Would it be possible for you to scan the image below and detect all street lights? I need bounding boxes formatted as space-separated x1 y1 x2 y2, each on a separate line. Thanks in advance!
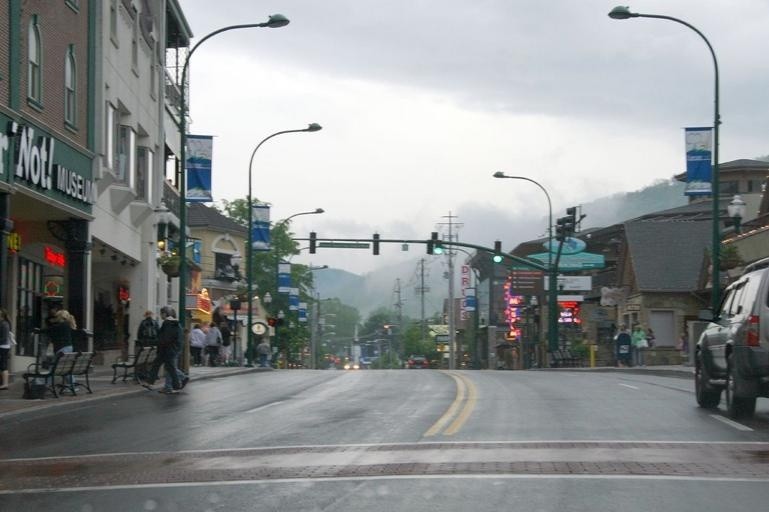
605 4 723 318
728 194 746 234
245 122 323 366
180 12 292 375
302 264 327 275
384 323 401 329
277 208 326 360
492 171 556 360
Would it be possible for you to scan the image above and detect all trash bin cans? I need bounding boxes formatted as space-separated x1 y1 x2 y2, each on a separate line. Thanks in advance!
259 354 267 367
614 332 631 362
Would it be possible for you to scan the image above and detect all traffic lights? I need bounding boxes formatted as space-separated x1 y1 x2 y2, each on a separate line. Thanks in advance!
555 217 566 241
493 242 504 264
565 207 574 232
435 240 442 255
268 317 275 325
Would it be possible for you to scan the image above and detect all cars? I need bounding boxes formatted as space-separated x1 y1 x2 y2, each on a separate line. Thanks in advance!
345 361 359 370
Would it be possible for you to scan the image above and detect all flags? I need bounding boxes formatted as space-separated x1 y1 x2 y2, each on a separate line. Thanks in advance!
466 287 476 313
278 263 292 292
683 126 713 197
185 135 212 202
288 288 300 311
251 206 271 251
298 304 307 322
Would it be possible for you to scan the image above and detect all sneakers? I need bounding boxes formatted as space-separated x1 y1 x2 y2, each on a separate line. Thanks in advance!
141 383 153 390
158 377 189 393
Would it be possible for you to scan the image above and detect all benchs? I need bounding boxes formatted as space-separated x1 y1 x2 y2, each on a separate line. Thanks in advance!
22 351 95 399
111 345 158 385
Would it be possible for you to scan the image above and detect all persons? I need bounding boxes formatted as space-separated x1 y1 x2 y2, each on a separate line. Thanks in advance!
610 322 688 369
43 300 233 392
257 338 270 367
1 308 17 389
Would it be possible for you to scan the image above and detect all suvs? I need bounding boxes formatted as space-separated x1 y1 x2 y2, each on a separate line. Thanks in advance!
407 353 429 370
692 256 768 415
